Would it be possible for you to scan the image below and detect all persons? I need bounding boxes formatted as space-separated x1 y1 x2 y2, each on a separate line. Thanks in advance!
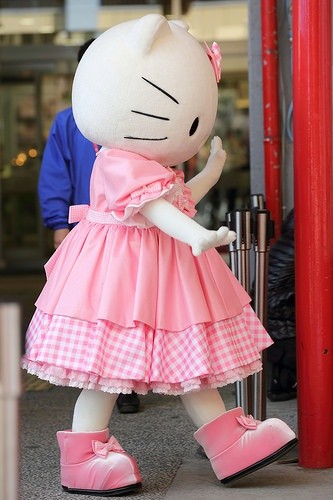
38 38 142 410
249 209 297 401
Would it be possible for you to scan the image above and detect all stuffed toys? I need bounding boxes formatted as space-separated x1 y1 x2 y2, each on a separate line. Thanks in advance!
19 13 299 496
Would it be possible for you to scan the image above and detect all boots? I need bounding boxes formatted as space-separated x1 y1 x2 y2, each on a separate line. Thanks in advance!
194 406 296 484
56 429 140 492
